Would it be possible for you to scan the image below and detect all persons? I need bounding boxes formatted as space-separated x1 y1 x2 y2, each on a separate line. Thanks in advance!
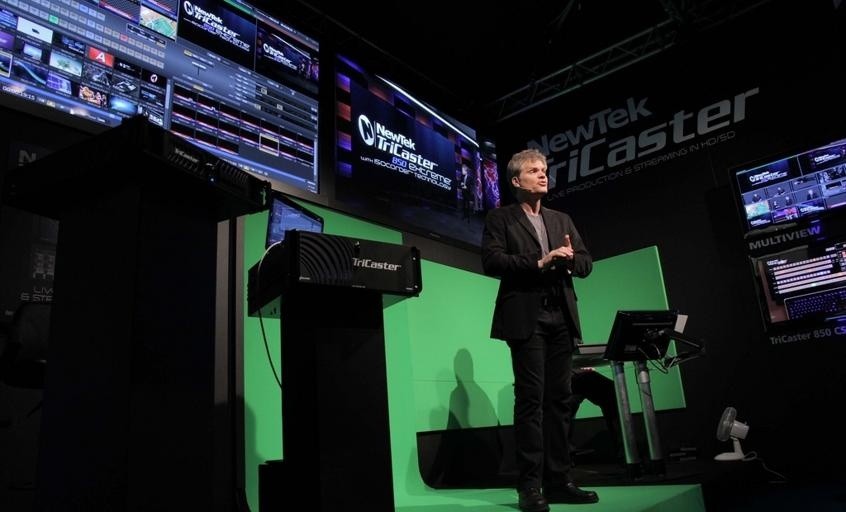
772 200 780 209
785 197 791 206
751 193 765 203
480 147 600 511
457 163 476 226
807 188 819 200
774 186 787 195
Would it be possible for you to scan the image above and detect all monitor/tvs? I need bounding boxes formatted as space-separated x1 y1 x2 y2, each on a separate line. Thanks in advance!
1 1 505 256
727 136 846 232
744 219 845 359
604 308 678 360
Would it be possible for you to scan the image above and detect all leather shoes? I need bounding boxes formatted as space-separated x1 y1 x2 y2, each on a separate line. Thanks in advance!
518 489 550 512
550 482 599 504
